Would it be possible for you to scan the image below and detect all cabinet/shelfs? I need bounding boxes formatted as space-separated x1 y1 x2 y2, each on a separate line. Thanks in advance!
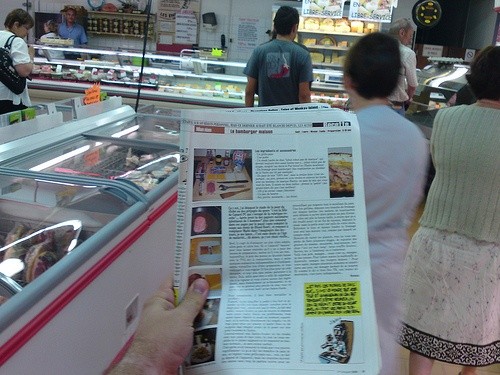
85 10 155 41
283 8 383 75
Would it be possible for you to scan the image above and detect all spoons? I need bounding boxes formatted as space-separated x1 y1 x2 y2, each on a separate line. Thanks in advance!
220 185 244 190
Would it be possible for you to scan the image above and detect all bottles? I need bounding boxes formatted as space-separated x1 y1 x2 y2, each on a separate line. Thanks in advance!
223 151 230 166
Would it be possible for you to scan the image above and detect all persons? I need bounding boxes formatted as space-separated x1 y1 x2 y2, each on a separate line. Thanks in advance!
389 18 419 118
242 6 314 106
106 278 209 375
58 8 88 60
0 8 38 115
258 30 310 52
343 32 429 375
393 46 500 375
36 20 65 61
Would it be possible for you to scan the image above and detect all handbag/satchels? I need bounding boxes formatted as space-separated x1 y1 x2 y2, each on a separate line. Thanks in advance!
0 34 27 95
